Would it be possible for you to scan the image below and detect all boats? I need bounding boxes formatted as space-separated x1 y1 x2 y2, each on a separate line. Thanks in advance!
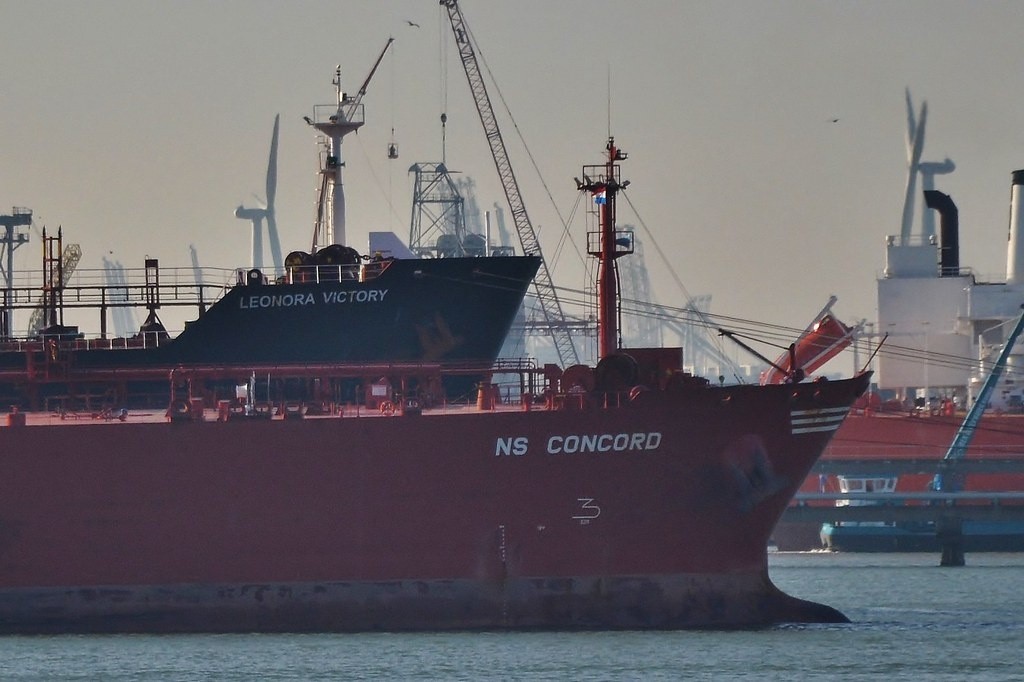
761 86 1024 554
0 35 876 636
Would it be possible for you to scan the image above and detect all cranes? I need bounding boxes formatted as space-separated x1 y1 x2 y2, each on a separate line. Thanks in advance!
444 1 581 370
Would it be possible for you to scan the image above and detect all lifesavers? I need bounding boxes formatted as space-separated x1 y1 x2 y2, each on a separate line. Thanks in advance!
380 401 396 417
